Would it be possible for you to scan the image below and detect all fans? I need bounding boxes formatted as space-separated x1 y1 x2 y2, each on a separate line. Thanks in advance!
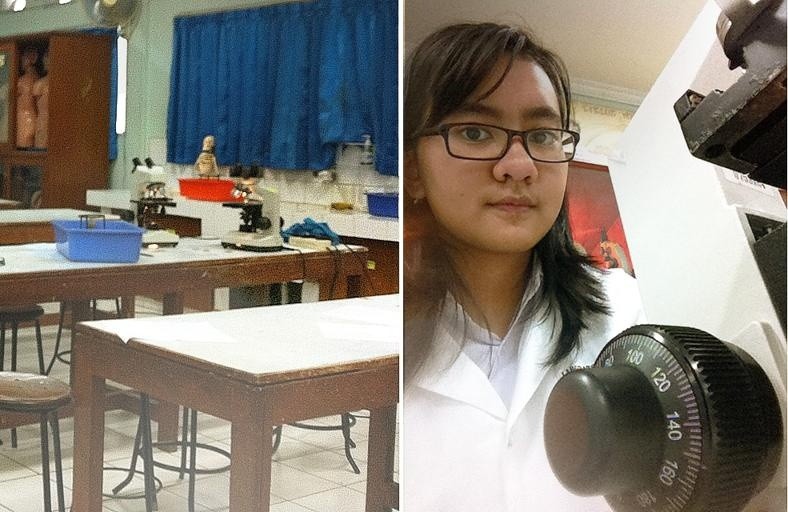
82 0 139 29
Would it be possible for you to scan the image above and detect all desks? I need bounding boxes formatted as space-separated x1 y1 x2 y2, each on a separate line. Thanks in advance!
0 190 399 512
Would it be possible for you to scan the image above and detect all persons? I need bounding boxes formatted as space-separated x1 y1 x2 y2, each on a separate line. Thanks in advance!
402 20 695 512
32 53 51 150
16 49 41 150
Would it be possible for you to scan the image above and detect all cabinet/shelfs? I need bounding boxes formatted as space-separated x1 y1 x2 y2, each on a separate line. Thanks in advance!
1 29 112 211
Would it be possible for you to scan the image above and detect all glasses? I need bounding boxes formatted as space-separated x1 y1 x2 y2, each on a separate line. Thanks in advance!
414 122 580 163
